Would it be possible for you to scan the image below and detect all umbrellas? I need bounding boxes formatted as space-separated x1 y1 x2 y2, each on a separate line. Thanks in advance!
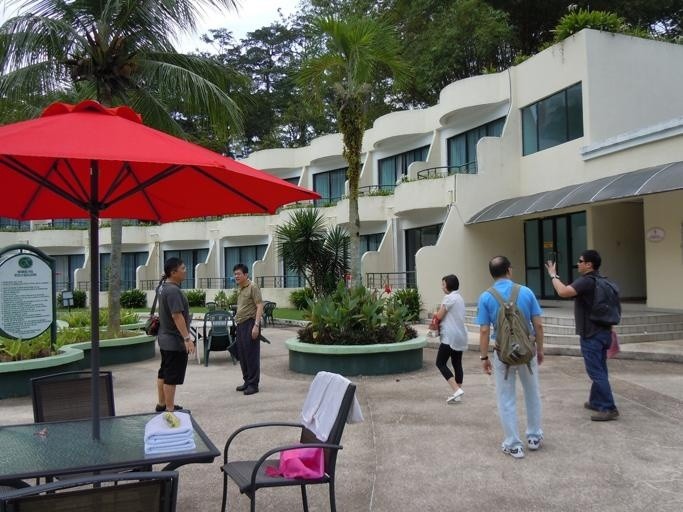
0 98 321 441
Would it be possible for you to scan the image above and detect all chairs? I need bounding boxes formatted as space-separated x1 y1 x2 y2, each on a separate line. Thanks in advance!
0 471 179 512
221 370 357 512
31 370 118 495
190 327 200 364
207 301 276 327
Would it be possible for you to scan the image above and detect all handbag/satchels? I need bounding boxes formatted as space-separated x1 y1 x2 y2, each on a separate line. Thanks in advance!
425 316 439 338
144 317 159 335
226 340 239 361
606 331 618 358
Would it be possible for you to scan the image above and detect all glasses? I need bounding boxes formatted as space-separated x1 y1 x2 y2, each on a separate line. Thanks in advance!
578 259 589 263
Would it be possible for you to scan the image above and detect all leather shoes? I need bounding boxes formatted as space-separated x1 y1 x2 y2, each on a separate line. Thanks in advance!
590 409 619 421
244 388 258 395
236 385 247 391
583 402 599 411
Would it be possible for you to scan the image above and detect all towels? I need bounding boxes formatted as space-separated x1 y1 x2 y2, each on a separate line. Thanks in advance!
144 411 195 455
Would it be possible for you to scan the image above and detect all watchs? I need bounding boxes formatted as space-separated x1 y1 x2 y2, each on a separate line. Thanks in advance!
480 356 488 360
551 274 560 280
183 336 191 342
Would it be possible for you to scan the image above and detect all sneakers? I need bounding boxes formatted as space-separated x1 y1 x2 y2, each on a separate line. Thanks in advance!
445 388 463 402
527 438 539 450
173 409 190 413
454 396 460 402
501 442 524 459
156 404 182 412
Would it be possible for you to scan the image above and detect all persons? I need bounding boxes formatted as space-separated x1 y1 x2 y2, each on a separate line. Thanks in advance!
471 255 545 458
155 257 194 412
233 263 263 396
544 249 620 421
432 274 469 403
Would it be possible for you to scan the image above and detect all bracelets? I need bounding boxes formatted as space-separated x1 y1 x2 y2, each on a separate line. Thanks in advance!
255 319 261 327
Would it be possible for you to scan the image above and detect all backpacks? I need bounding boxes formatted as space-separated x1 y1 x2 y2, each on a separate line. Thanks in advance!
580 275 620 325
486 283 536 380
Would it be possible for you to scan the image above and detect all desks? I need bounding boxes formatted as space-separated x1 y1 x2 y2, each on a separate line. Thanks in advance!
0 410 220 494
203 310 237 367
